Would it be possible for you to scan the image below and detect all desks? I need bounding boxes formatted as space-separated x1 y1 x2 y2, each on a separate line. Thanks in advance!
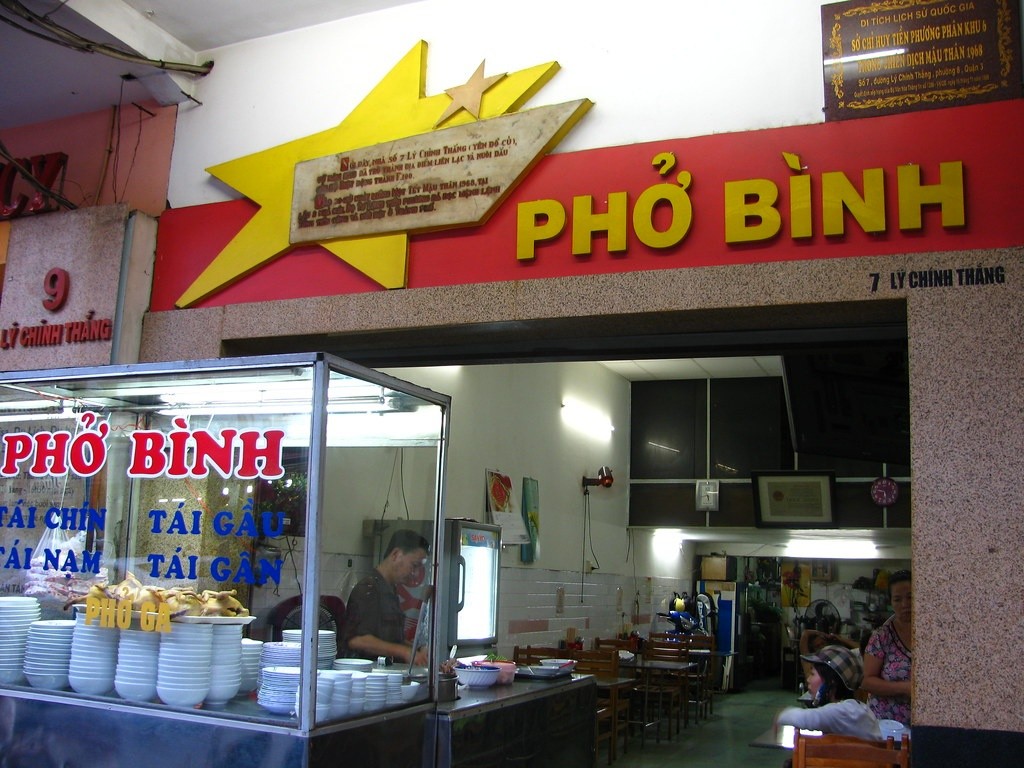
747 724 824 751
652 647 739 717
590 658 696 749
796 690 815 709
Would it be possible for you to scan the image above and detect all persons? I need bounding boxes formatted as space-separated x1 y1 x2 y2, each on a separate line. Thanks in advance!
339 530 427 666
772 570 912 768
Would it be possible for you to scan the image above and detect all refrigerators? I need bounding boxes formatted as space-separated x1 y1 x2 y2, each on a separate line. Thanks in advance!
372 514 502 657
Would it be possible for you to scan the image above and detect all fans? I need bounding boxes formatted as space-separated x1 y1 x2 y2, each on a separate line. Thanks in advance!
803 599 842 634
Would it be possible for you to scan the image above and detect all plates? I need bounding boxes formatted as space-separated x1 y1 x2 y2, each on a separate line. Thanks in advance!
257 629 374 714
880 719 911 741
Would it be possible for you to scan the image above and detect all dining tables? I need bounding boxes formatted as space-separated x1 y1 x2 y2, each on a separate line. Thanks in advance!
592 677 636 766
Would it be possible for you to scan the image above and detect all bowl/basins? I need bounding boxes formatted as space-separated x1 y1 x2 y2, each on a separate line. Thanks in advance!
440 673 459 701
297 669 420 723
452 666 502 689
527 665 560 676
0 597 263 707
539 658 578 672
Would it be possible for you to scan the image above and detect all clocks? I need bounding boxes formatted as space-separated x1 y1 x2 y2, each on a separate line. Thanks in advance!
869 477 900 508
695 479 719 511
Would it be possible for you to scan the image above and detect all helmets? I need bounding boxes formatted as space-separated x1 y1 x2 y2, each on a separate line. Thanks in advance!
800 645 864 692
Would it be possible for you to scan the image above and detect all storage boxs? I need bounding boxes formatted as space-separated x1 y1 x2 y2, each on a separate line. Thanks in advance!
701 556 736 582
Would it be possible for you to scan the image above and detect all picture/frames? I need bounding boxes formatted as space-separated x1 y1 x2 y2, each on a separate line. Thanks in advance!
750 470 839 529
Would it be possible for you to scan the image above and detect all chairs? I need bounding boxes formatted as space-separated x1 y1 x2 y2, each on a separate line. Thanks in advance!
511 630 911 768
